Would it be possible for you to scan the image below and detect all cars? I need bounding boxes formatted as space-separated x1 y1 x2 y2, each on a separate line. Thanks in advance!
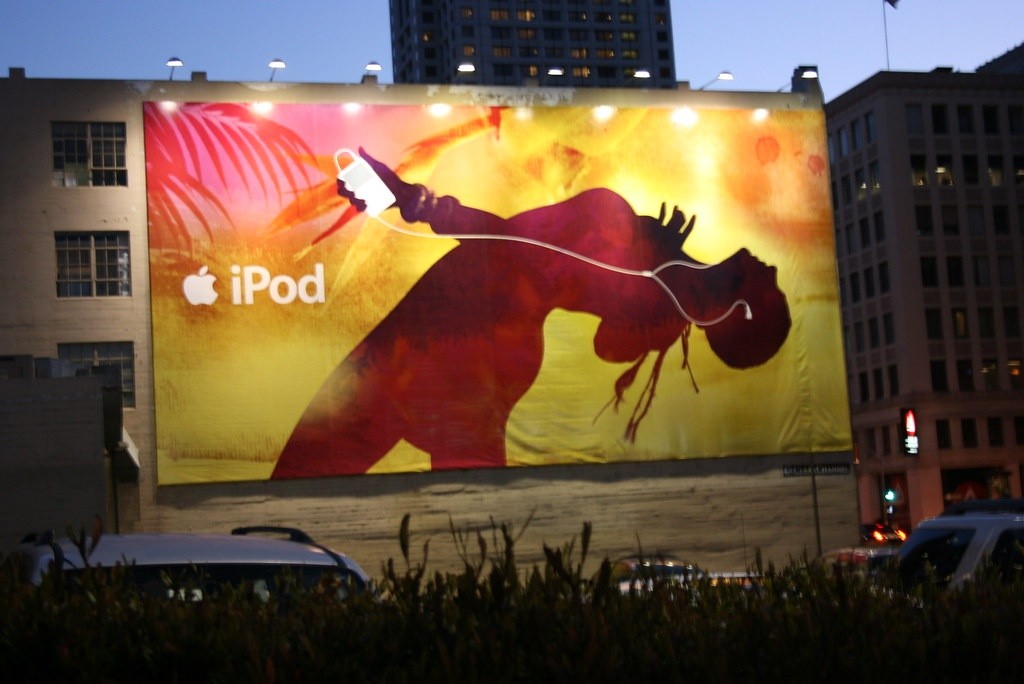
591 555 708 598
820 546 901 574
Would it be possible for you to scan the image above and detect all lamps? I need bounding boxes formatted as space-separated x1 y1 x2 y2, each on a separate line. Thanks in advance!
452 61 476 84
699 70 733 91
268 58 286 82
540 66 565 87
166 57 184 81
365 61 382 75
618 68 651 88
778 71 818 93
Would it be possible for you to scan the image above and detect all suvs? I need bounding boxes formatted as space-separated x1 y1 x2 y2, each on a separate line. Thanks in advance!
8 525 379 612
891 498 1024 599
